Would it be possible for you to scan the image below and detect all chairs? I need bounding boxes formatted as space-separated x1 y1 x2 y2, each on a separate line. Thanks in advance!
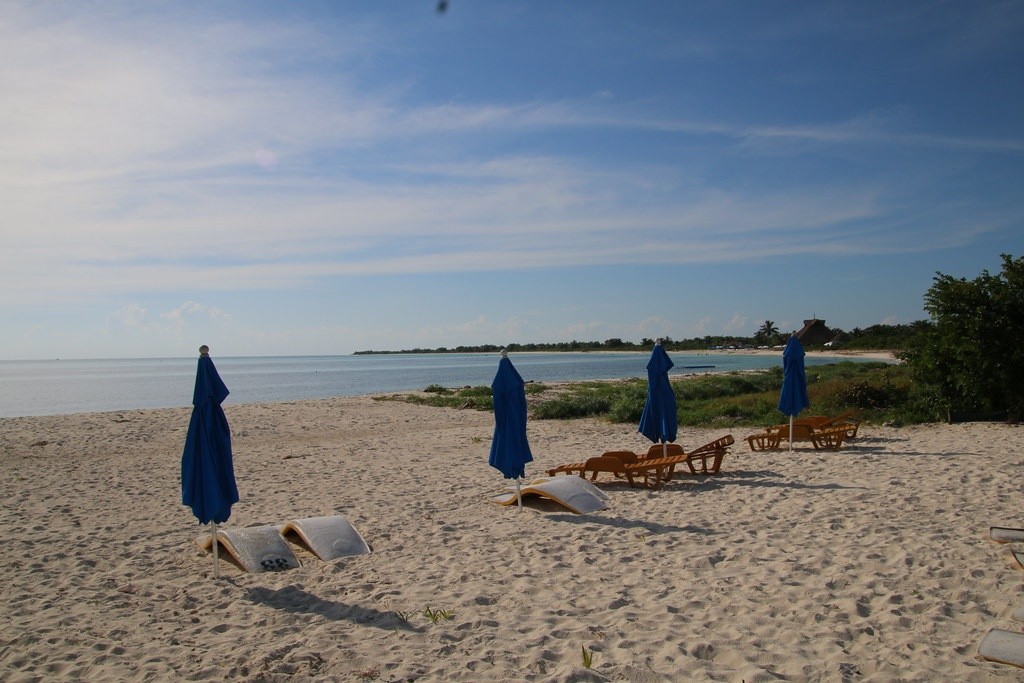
613 434 735 482
744 423 858 452
764 407 863 444
544 450 687 491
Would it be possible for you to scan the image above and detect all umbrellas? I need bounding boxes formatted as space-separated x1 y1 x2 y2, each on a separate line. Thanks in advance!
180 345 240 579
488 349 534 511
777 330 811 451
637 337 678 475
